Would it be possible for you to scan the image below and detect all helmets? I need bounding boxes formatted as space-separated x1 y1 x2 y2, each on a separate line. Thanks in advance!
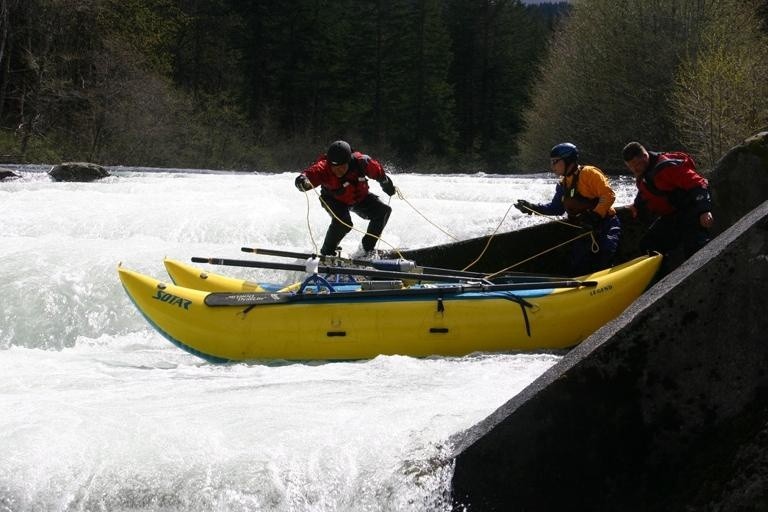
327 141 352 165
549 143 579 165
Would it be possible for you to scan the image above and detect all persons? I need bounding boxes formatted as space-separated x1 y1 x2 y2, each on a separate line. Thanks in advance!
514 142 623 271
621 141 715 264
295 139 395 258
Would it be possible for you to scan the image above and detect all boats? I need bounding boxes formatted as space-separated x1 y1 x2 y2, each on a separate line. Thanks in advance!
116 248 665 365
162 256 483 292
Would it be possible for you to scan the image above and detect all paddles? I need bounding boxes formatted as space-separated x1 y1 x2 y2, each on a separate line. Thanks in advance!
203 281 597 307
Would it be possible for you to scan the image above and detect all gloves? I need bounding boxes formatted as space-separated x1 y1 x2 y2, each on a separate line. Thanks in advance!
295 175 311 191
513 199 533 216
586 211 602 225
380 175 395 196
